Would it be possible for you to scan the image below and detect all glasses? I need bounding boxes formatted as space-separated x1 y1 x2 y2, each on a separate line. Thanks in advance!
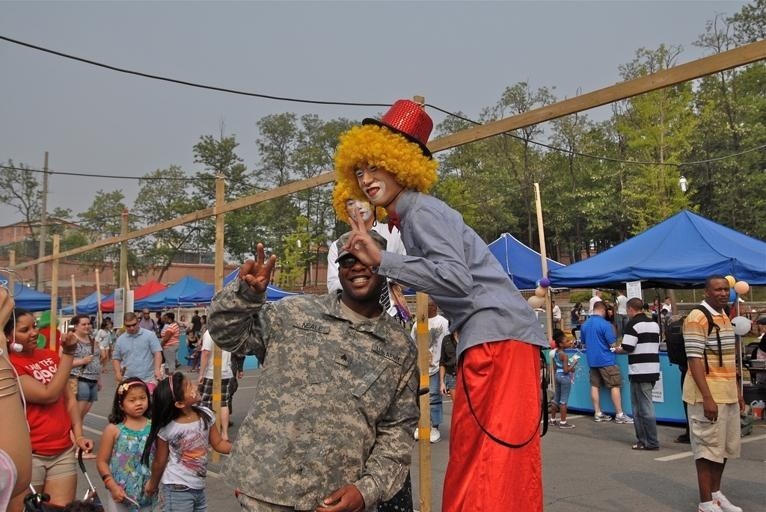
124 320 138 328
337 258 357 268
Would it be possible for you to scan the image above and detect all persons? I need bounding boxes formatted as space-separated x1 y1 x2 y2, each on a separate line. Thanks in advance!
207 228 423 512
409 288 765 451
325 181 414 511
332 97 552 511
0 281 246 511
680 275 746 511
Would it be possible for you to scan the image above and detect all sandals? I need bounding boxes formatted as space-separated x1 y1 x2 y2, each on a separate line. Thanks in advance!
631 441 644 450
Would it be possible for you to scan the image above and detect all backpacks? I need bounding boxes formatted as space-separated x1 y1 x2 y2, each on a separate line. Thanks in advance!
666 305 713 369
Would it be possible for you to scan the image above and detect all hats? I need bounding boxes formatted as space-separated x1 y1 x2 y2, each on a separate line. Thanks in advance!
363 100 433 160
335 230 387 264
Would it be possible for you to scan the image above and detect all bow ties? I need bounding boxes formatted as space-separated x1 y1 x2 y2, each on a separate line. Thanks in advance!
386 210 401 233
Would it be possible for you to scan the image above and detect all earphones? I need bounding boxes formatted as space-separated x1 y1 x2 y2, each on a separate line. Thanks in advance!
10 342 24 352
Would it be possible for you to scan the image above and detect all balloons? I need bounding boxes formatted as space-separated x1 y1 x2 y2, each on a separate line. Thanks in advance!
540 277 551 288
724 275 736 288
734 280 750 295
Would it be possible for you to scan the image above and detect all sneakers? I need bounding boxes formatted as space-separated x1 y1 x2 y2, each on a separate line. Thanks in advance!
594 412 634 424
715 495 743 512
550 420 575 429
698 500 723 512
75 448 96 459
413 427 441 443
